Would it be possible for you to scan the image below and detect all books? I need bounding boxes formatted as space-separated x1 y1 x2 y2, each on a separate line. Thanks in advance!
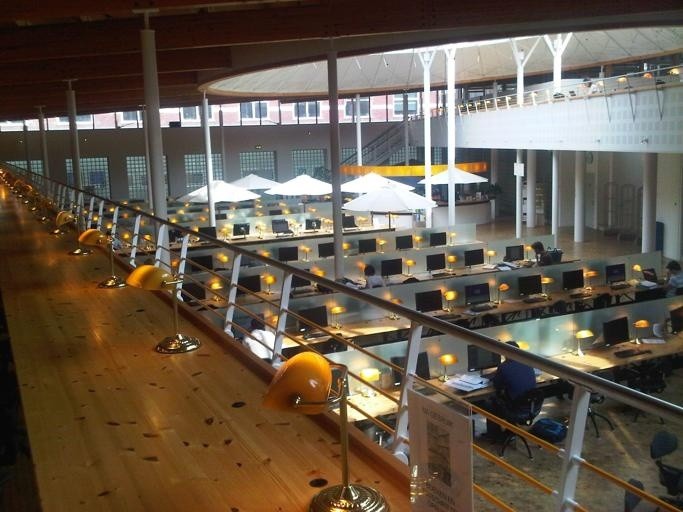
635 280 658 289
458 374 489 385
481 261 522 272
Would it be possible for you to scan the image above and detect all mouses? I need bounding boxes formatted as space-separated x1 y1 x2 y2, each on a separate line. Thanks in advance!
645 349 651 353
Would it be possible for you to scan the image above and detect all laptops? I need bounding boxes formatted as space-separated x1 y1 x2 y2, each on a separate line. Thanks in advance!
642 268 663 284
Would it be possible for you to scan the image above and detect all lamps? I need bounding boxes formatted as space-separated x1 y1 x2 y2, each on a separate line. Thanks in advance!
260 348 392 512
433 58 682 115
0 167 201 354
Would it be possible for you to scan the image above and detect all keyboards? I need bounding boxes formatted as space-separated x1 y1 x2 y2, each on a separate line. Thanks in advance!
415 388 436 396
614 347 643 358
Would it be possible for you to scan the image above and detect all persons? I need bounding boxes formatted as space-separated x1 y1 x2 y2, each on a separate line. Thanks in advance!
362 265 388 287
660 260 682 295
238 314 274 363
476 339 538 442
531 240 553 268
583 77 602 95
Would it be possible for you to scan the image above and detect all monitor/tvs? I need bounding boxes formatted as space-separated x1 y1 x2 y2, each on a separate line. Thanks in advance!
332 369 349 394
603 316 629 346
467 344 501 375
670 306 683 331
391 351 430 386
635 288 665 303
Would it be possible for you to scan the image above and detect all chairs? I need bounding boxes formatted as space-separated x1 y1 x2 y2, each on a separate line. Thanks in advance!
623 477 682 512
488 384 545 460
563 386 616 437
646 429 683 498
